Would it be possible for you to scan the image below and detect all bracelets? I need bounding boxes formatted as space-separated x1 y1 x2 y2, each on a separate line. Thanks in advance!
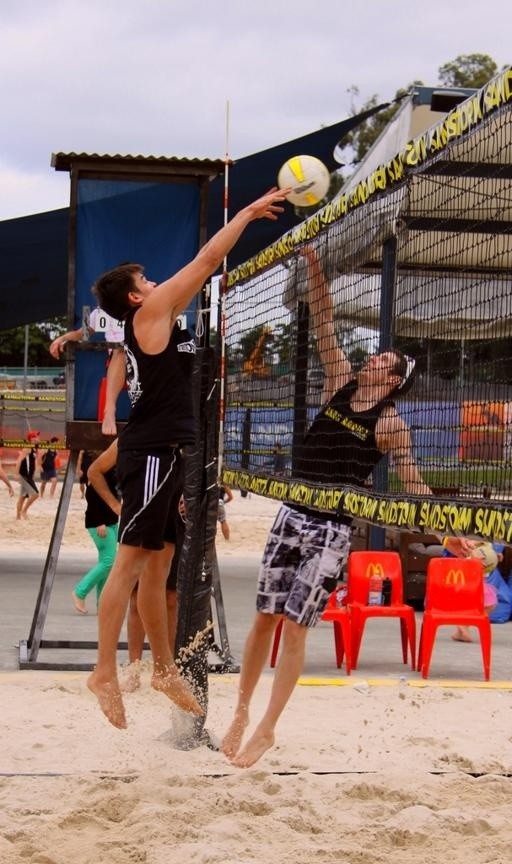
443 534 448 547
439 534 446 543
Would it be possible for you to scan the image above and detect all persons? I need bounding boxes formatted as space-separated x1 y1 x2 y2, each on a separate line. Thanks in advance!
219 481 235 502
14 432 40 523
261 440 288 476
216 498 229 541
88 427 187 692
1 467 14 499
87 181 293 731
222 244 474 771
76 448 88 496
38 435 62 500
70 464 121 614
442 530 512 644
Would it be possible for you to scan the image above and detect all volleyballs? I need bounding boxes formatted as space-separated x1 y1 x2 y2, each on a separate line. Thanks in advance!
278 155 330 207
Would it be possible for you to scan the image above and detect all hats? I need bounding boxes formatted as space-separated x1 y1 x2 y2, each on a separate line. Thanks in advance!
27 432 40 439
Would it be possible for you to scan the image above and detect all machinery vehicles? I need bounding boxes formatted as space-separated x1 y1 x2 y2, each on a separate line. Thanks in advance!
235 323 275 380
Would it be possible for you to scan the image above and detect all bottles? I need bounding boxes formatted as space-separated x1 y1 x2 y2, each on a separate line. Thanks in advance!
367 569 383 606
382 576 392 606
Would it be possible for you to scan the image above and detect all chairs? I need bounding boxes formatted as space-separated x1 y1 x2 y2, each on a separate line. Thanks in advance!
271 550 495 685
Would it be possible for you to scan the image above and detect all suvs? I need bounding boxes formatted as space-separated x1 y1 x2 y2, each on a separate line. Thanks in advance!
306 367 326 388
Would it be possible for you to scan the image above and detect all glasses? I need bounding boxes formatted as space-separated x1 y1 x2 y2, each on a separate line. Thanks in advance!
398 354 415 389
